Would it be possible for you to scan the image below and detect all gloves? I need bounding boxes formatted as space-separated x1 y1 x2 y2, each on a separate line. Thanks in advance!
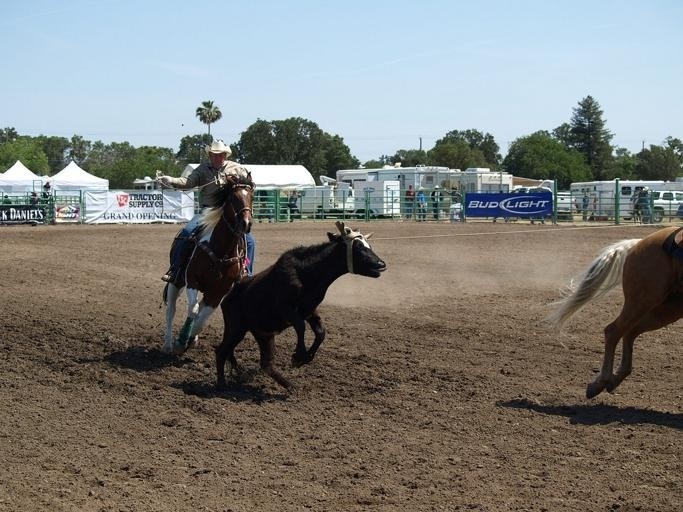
157 176 173 189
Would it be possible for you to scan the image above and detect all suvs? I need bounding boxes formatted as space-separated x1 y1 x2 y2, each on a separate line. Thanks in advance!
629 190 683 222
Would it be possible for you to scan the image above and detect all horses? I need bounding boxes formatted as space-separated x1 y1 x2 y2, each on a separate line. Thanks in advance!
160 167 257 358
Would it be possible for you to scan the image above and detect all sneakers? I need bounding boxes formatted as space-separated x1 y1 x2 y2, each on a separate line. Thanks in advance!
163 269 178 283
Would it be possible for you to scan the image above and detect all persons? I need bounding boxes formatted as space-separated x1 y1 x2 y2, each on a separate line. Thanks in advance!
31 195 39 226
258 203 272 223
2 194 11 205
288 191 304 222
405 185 462 222
163 142 255 282
630 187 653 224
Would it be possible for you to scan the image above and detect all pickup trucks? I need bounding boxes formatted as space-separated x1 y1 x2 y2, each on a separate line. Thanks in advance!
508 185 577 220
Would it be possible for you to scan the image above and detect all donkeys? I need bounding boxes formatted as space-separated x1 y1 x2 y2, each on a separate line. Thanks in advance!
534 224 683 398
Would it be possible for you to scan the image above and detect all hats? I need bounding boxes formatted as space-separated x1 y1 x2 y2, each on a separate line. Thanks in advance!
408 184 457 191
205 139 232 159
643 187 649 192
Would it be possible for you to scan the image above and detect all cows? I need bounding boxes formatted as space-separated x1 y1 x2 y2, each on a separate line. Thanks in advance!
213 218 389 392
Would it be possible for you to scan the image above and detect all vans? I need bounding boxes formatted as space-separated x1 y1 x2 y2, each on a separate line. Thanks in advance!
294 185 355 219
